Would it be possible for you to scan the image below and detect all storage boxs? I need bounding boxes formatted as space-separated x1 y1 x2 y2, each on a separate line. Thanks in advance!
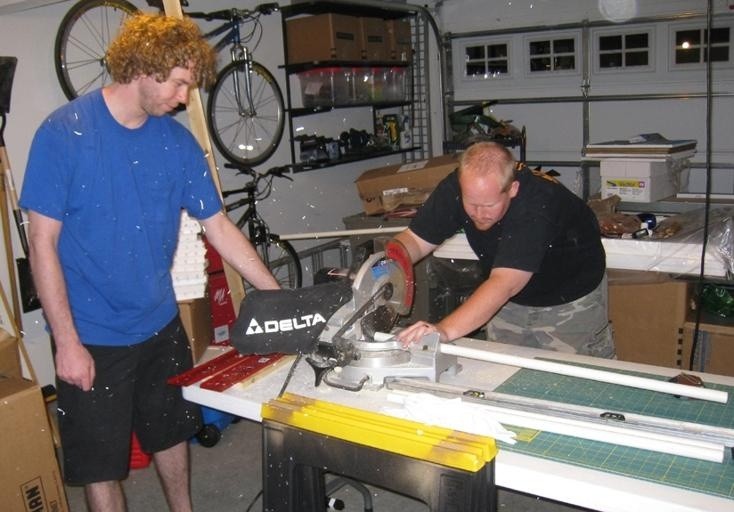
354 153 464 216
286 12 413 108
0 377 69 512
175 283 214 365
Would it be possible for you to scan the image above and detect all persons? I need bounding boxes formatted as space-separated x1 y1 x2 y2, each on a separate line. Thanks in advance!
378 141 620 361
18 8 283 512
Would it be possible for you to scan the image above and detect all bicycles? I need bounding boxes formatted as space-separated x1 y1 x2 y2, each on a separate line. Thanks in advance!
221 160 303 291
53 0 285 166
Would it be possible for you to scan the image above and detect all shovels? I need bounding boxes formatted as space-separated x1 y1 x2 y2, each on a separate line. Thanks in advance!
0 136 44 314
0 55 26 333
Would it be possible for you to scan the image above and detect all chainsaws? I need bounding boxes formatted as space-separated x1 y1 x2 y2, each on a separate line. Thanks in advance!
309 239 414 387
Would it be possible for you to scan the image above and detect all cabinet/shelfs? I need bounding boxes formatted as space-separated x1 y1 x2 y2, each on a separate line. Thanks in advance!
277 0 421 173
